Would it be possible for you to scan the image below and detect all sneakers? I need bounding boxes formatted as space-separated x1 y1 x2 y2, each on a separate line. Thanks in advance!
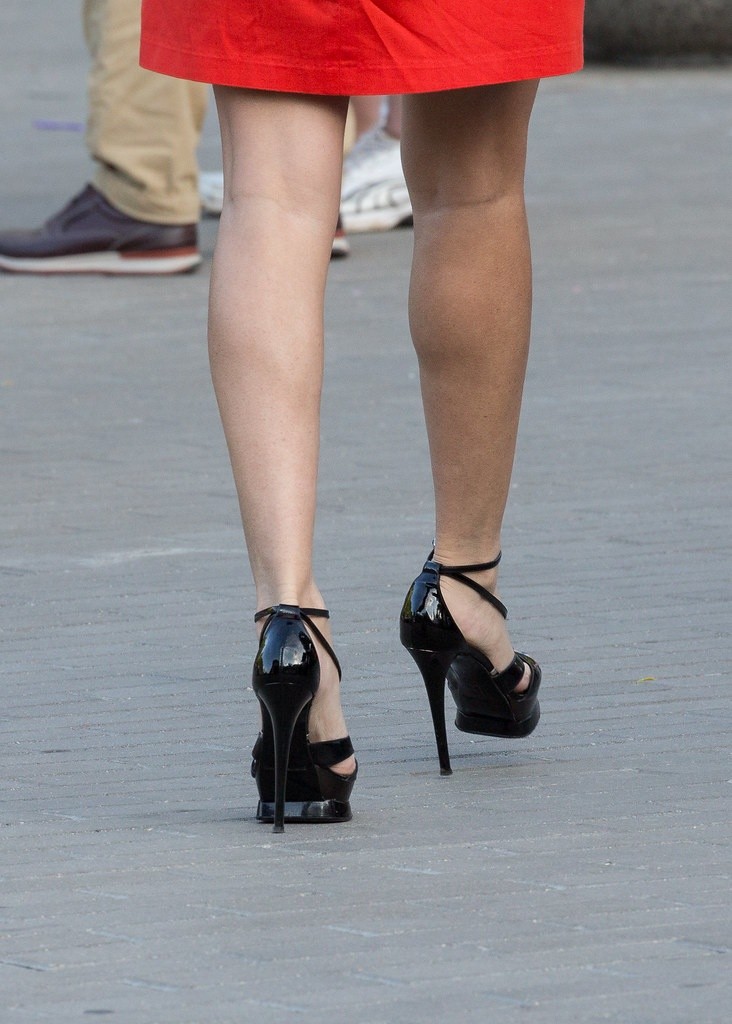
338 125 416 235
330 214 352 254
199 171 227 215
0 183 202 276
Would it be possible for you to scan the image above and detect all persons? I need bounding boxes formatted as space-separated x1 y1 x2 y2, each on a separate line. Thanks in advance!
0 0 349 273
139 0 586 833
199 95 413 233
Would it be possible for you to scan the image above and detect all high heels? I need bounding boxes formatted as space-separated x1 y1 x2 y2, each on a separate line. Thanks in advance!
252 602 361 834
399 546 541 777
